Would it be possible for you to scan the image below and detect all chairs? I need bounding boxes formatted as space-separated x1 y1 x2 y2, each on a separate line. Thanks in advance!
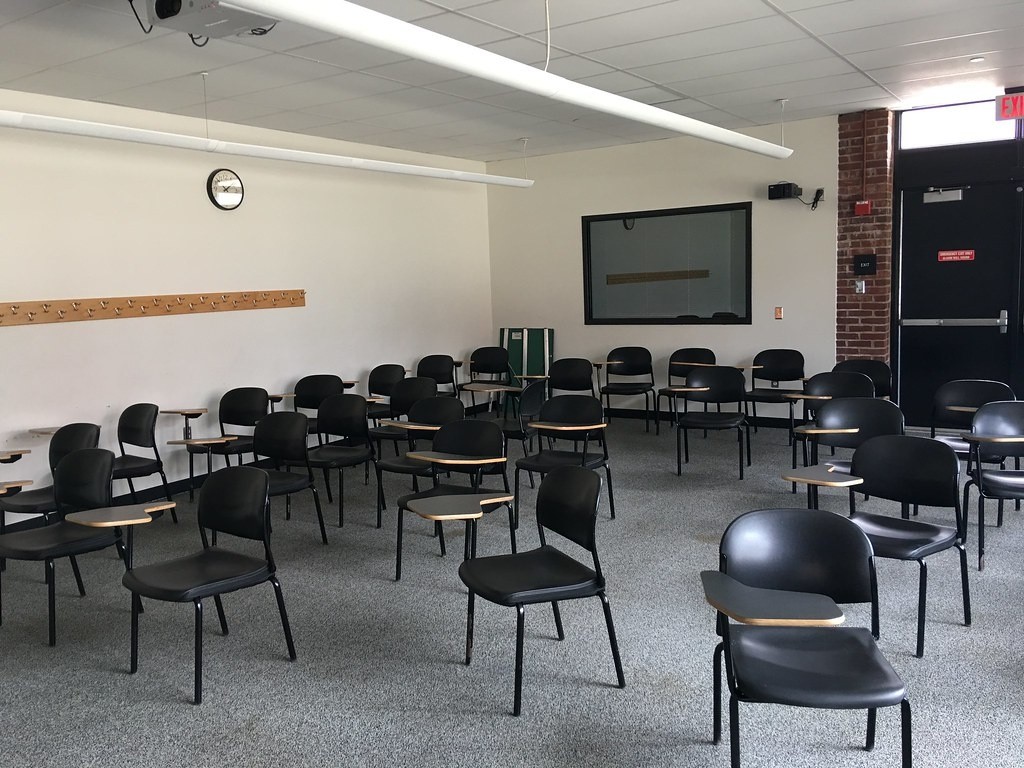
0 346 1024 581
66 465 295 703
700 507 912 768
0 447 144 645
407 466 626 717
776 434 972 658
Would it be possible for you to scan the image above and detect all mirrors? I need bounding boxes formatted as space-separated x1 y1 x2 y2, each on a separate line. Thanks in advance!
580 201 752 325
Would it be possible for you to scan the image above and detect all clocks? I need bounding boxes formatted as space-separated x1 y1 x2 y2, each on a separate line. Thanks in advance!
207 168 244 210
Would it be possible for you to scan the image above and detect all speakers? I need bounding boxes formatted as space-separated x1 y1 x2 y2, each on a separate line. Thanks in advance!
767 183 798 200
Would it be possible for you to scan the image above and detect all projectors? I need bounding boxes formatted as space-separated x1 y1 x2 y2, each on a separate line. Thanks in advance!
145 0 280 39
